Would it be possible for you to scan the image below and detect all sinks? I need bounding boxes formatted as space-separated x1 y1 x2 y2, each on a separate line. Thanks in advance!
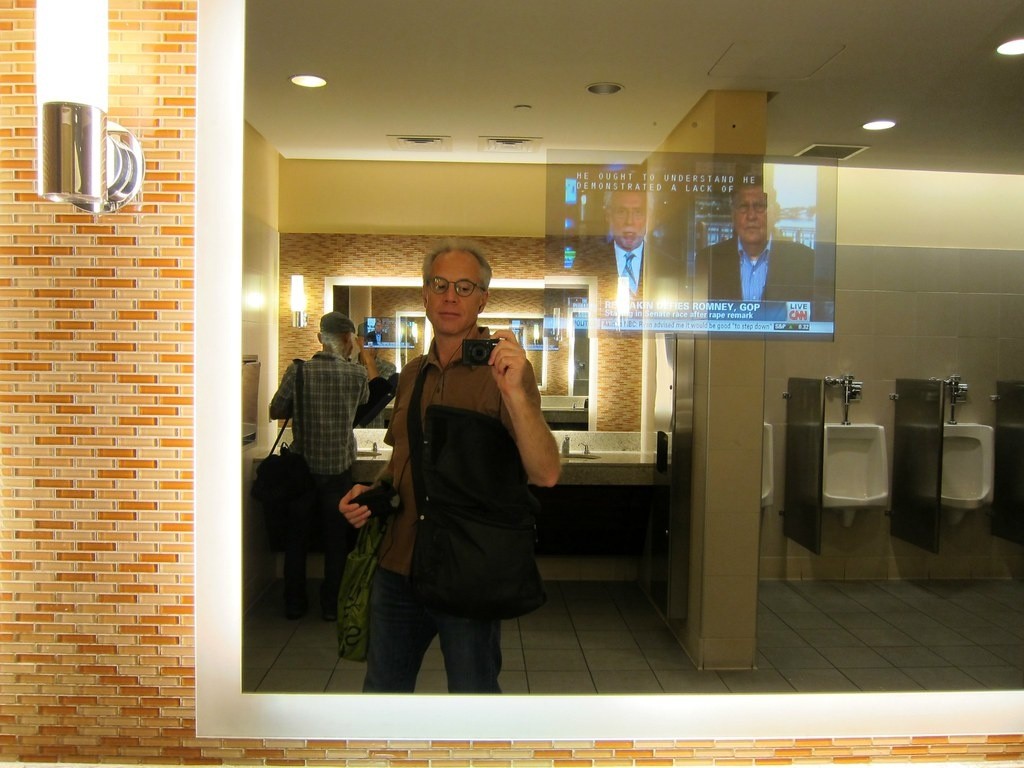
558 454 602 461
356 451 382 457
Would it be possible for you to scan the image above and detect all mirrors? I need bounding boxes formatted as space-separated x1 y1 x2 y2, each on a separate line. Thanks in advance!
322 276 598 433
194 1 1024 739
395 309 550 392
480 324 527 358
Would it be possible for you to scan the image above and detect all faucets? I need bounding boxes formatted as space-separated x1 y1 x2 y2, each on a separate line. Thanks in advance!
560 436 571 456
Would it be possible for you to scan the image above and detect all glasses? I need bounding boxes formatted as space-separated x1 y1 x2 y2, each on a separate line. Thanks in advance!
427 276 484 298
736 201 767 213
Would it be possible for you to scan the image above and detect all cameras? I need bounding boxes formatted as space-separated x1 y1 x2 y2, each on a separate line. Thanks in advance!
461 339 501 365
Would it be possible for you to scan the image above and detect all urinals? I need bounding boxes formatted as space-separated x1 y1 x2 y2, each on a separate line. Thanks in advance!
822 375 890 527
927 374 995 526
761 421 774 509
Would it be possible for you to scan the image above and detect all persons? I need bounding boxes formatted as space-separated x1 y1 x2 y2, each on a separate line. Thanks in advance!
355 323 395 428
694 177 812 303
401 324 416 344
572 187 676 299
367 318 390 342
339 242 559 694
270 312 378 622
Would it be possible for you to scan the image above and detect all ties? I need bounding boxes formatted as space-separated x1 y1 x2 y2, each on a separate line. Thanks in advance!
623 252 636 291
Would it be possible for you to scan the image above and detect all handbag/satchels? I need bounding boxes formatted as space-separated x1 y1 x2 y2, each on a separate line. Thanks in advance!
337 475 393 660
411 492 547 619
251 448 322 549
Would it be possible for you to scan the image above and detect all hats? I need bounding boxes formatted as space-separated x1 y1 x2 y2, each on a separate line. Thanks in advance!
320 312 356 334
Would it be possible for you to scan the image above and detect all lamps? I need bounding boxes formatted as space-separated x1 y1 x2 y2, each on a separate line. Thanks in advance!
533 323 541 346
32 0 145 212
290 273 308 328
